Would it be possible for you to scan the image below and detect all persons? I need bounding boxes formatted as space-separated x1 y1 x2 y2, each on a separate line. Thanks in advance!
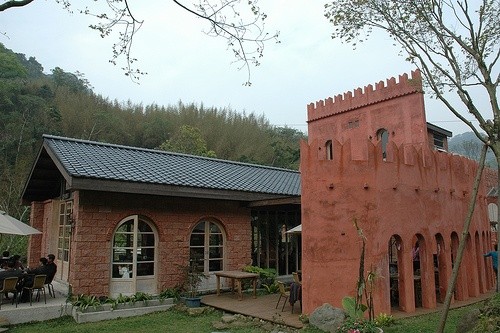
122 264 132 279
483 243 500 292
413 241 420 272
0 250 57 302
288 242 295 256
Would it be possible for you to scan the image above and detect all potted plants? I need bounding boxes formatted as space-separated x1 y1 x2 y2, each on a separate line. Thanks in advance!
176 261 207 308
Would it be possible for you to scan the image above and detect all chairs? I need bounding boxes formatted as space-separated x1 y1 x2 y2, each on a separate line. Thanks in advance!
0 276 19 309
292 272 300 283
19 275 48 306
276 280 292 313
37 277 56 300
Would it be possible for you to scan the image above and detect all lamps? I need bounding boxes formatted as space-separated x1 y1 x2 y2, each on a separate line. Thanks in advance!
67 214 74 234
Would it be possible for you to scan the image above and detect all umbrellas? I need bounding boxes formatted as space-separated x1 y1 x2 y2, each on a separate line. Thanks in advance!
285 224 302 232
0 209 43 235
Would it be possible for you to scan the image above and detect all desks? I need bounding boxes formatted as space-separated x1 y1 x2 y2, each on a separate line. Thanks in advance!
215 272 261 302
